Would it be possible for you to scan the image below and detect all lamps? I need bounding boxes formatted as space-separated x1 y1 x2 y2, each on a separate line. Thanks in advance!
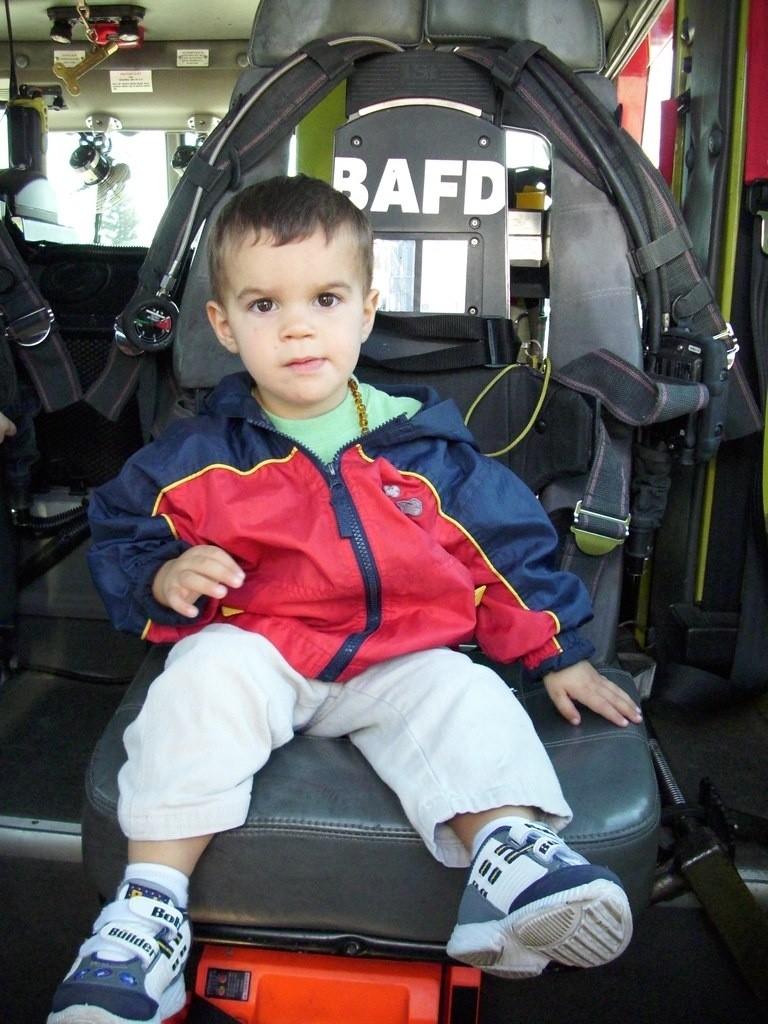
118 4 145 42
47 6 73 44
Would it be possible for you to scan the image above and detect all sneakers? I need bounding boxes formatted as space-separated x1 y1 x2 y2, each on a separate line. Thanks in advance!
446 821 633 978
45 878 194 1024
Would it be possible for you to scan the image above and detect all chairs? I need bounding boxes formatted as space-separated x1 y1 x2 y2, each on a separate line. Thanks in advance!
81 0 661 967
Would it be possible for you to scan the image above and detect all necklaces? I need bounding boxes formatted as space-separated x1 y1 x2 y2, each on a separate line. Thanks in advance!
348 377 369 435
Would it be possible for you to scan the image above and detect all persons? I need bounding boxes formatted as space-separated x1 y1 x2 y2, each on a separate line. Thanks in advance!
41 174 643 1024
